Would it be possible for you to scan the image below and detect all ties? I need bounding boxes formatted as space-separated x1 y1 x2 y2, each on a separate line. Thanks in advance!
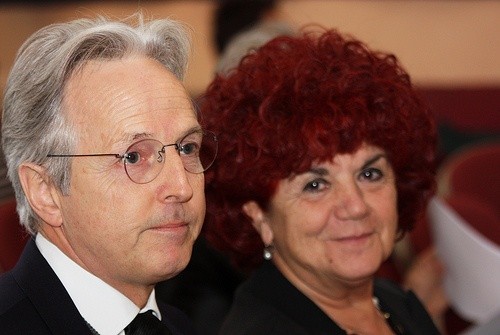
123 310 172 335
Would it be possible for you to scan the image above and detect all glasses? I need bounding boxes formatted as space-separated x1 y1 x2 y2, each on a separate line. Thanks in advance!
46 131 220 185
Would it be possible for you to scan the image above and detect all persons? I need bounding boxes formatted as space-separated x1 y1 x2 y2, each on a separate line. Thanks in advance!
192 23 444 335
0 12 248 335
